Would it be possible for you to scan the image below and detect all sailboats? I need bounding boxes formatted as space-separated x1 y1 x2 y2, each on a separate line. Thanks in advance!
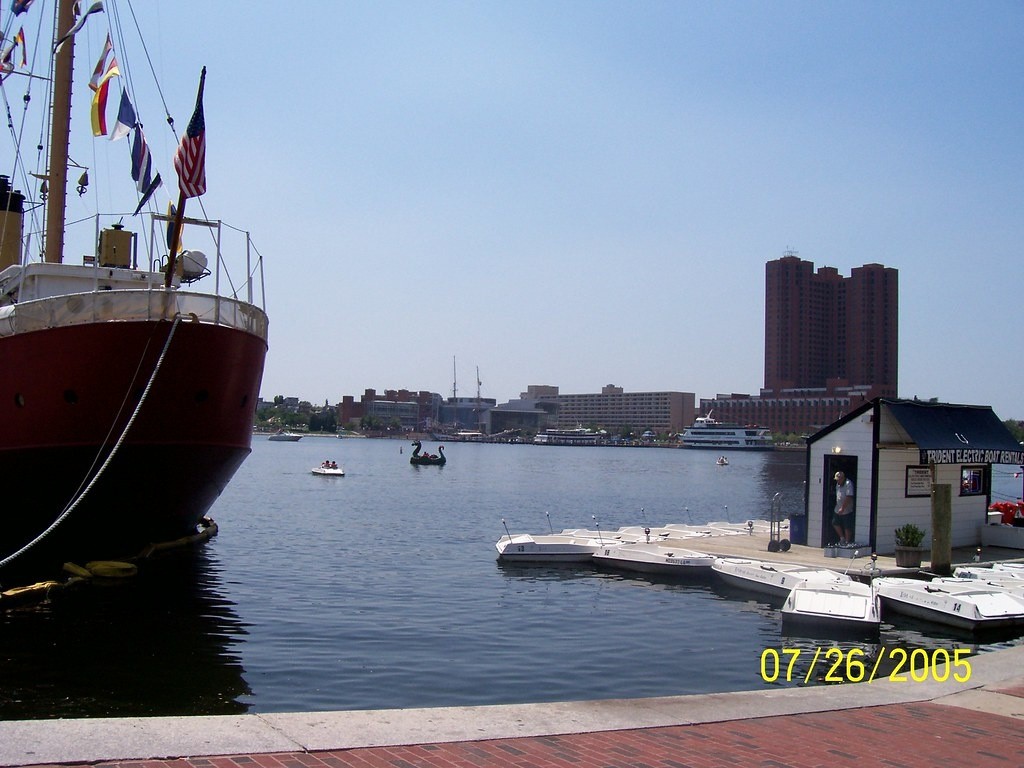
0 0 270 579
430 354 521 443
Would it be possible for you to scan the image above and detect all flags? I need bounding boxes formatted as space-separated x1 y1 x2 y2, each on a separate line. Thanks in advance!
11 0 35 16
174 99 207 199
88 32 120 136
110 86 163 217
0 27 27 71
54 0 103 54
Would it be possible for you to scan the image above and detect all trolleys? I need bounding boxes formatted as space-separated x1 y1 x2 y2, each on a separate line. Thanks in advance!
759 494 791 554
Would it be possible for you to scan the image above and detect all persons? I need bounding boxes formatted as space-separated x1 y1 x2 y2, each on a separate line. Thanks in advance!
423 452 429 457
832 471 854 545
322 460 338 469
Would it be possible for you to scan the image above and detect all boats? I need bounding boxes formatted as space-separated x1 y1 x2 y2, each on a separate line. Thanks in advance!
410 440 447 464
594 542 714 578
268 430 303 442
874 574 1023 632
561 519 790 545
781 571 881 638
494 534 596 562
535 429 603 444
312 466 343 476
713 556 804 599
679 408 775 450
799 411 843 445
717 457 728 465
